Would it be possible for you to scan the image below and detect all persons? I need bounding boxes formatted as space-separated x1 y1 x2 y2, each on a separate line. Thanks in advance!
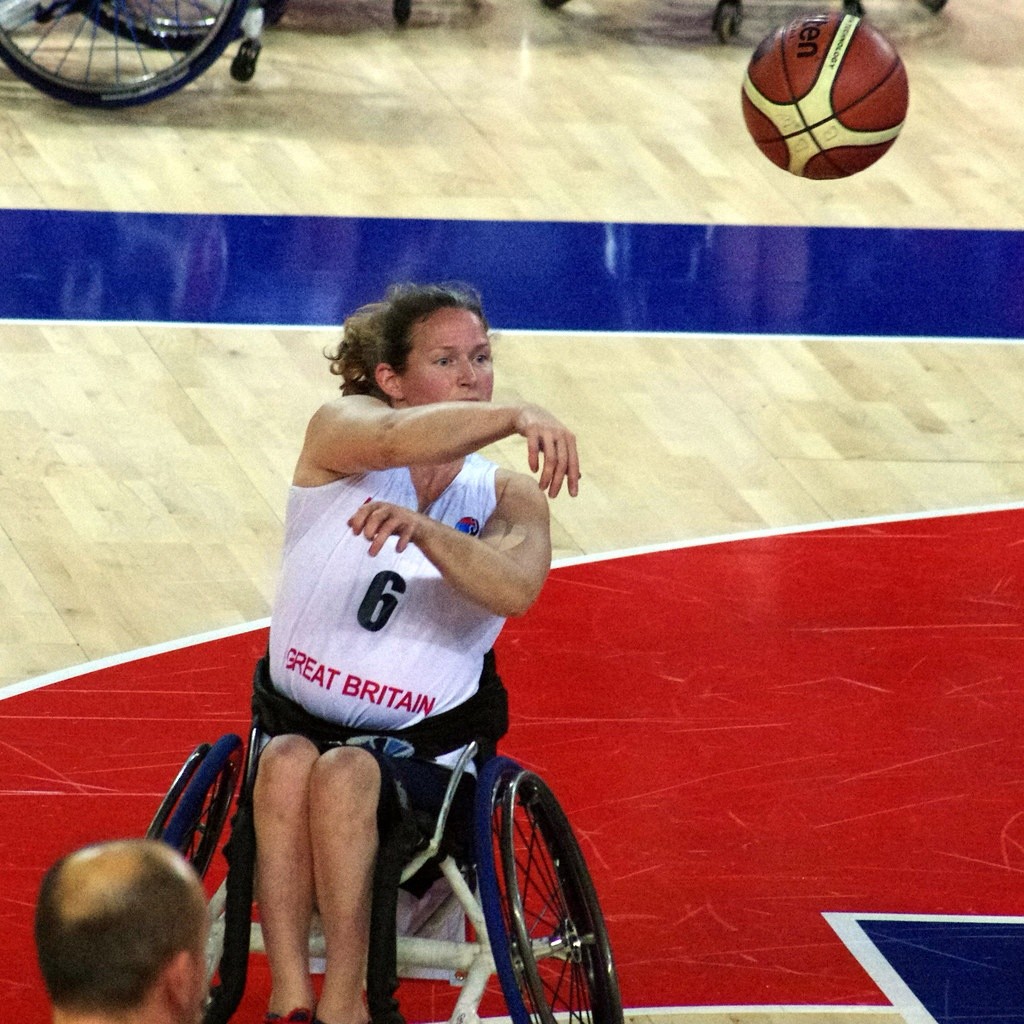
35 839 212 1024
253 284 582 1024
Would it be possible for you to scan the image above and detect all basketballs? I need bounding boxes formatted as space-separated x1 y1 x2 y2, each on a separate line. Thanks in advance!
738 11 912 184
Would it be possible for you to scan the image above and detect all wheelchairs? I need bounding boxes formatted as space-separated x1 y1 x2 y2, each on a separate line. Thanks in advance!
0 0 293 108
141 654 630 1024
